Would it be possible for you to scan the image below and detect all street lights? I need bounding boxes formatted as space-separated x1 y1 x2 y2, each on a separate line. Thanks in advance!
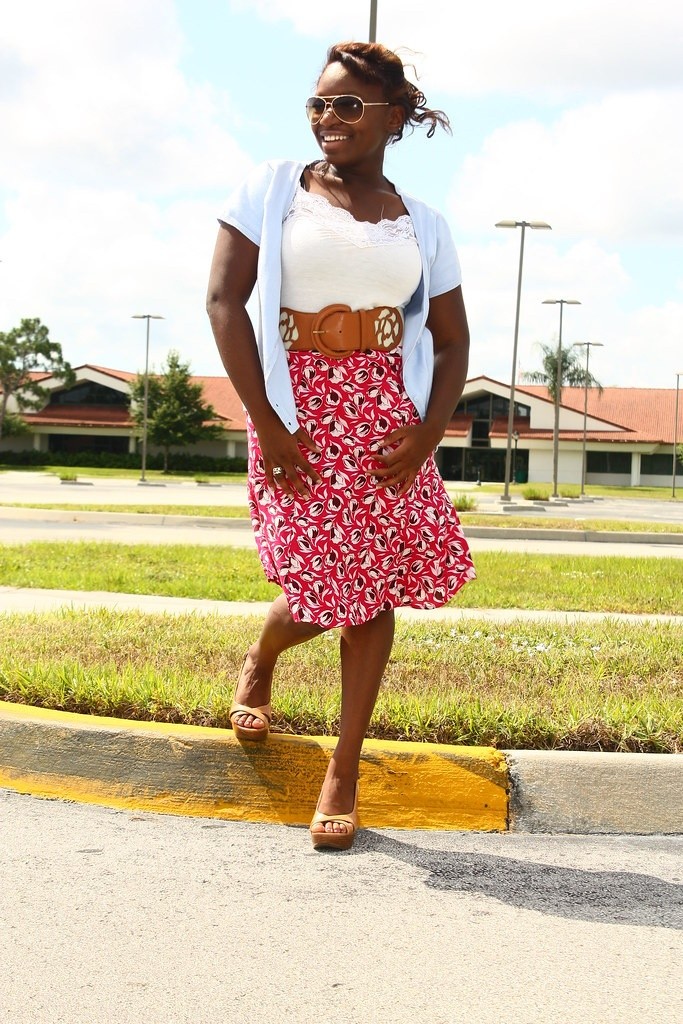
541 299 581 497
495 219 552 501
131 314 165 481
573 342 604 494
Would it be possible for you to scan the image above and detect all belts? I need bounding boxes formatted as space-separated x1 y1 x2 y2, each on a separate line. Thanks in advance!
278 303 406 360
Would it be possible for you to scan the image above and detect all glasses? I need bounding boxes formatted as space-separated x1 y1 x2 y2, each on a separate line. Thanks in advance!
305 93 394 126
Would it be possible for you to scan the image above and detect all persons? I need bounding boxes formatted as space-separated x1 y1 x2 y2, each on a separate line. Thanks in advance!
205 40 474 847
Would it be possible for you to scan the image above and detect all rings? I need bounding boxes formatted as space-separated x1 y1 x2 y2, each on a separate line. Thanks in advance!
272 466 284 478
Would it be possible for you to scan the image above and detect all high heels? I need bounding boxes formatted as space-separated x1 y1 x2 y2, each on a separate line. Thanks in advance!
230 651 273 741
309 779 361 849
